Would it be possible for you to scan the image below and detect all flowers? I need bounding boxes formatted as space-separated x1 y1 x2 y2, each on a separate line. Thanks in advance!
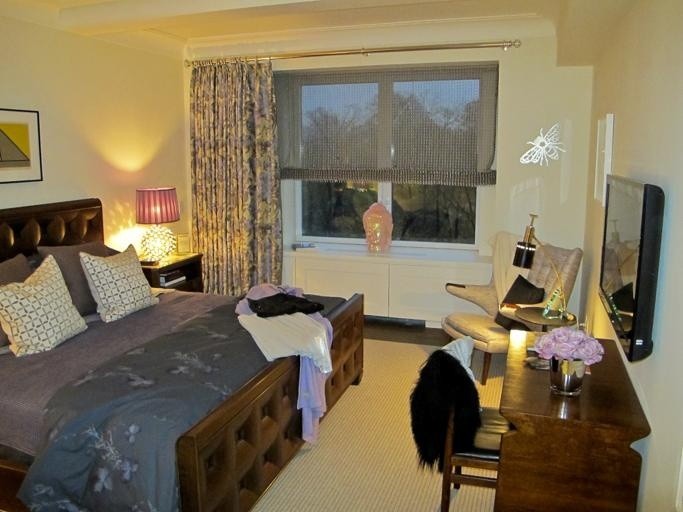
535 326 605 366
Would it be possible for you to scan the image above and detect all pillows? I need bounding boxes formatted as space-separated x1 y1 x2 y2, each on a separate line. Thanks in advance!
37 240 109 317
78 244 159 323
495 274 544 331
0 255 89 358
0 253 36 347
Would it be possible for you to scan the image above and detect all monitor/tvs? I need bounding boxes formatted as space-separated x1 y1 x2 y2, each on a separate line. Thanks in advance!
598 175 665 363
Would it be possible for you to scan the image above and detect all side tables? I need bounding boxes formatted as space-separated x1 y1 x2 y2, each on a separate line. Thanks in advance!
515 307 577 331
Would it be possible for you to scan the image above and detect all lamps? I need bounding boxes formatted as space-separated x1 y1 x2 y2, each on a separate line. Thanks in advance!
135 186 181 261
512 214 567 319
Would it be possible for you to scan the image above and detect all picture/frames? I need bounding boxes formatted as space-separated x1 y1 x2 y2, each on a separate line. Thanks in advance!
0 108 43 184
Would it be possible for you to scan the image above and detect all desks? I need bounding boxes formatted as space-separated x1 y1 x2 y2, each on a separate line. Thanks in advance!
493 328 651 512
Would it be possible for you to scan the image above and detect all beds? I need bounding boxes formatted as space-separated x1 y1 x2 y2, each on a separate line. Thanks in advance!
0 198 364 512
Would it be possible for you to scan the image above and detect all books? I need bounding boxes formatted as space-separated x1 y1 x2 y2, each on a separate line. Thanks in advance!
160 272 186 287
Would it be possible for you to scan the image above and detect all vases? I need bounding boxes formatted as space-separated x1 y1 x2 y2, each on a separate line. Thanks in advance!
550 359 584 395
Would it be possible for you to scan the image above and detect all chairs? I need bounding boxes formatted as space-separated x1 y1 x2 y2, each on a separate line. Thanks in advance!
433 336 509 512
441 231 583 385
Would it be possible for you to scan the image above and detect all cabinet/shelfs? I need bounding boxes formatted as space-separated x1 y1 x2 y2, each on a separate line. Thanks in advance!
140 252 204 292
